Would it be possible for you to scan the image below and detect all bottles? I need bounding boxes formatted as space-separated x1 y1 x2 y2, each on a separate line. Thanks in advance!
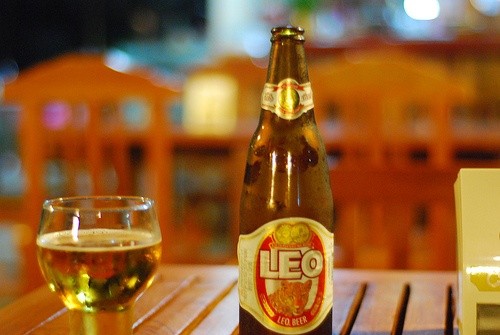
236 25 336 335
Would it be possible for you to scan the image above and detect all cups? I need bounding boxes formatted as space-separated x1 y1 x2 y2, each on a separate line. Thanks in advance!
36 194 161 311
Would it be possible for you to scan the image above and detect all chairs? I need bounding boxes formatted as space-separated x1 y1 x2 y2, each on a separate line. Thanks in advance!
1 52 182 280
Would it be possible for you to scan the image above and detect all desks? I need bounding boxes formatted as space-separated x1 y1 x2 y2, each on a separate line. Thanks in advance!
0 263 464 335
